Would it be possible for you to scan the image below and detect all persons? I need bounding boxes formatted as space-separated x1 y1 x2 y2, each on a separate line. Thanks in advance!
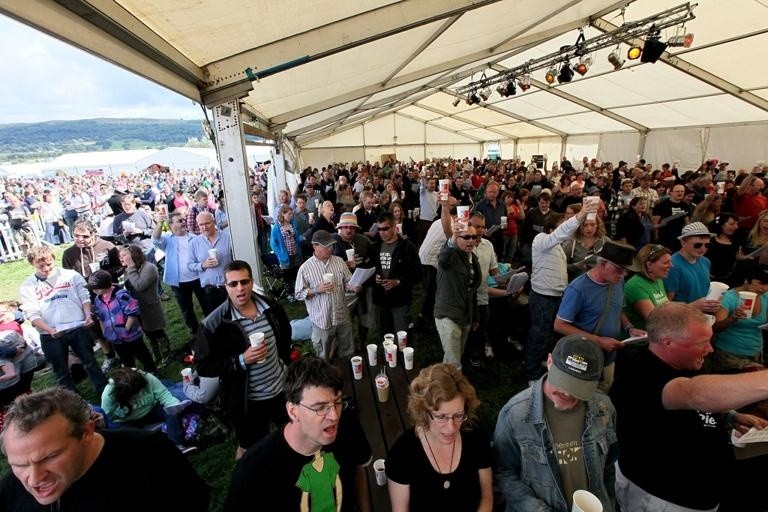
492 334 622 512
0 155 766 463
614 300 766 510
0 386 215 512
214 350 374 511
385 361 494 511
292 446 347 512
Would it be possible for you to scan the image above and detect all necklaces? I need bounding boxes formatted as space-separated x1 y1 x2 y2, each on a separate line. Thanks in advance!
425 433 457 491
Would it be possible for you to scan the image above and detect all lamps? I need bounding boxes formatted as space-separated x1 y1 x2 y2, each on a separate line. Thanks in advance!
451 19 696 108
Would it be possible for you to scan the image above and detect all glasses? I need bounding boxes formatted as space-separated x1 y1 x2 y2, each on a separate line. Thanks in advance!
376 226 392 231
173 217 187 223
307 186 313 188
459 234 478 241
475 224 485 228
298 399 349 416
226 278 254 287
426 406 468 423
693 243 714 249
73 234 90 238
626 185 632 187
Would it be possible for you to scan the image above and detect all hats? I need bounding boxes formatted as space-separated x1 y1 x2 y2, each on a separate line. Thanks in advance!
639 173 650 180
678 222 717 240
335 212 360 229
619 161 627 168
83 270 111 289
586 186 600 196
718 160 729 166
312 230 338 247
596 239 642 272
548 334 605 402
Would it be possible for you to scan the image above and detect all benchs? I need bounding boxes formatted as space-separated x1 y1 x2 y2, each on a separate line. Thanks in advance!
334 347 423 512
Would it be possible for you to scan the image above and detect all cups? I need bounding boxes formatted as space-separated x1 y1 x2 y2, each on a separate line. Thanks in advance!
248 332 265 364
413 207 419 220
375 373 390 402
421 166 425 174
180 368 193 388
366 345 376 366
584 196 600 220
89 261 101 276
344 249 354 263
400 191 404 199
499 216 507 229
407 210 411 218
314 199 318 208
208 249 219 265
322 273 333 293
396 225 402 234
438 178 448 201
717 182 723 193
737 291 758 320
705 280 727 303
357 164 362 171
308 213 313 222
571 490 603 512
159 205 168 219
373 460 385 486
456 205 469 232
350 354 363 382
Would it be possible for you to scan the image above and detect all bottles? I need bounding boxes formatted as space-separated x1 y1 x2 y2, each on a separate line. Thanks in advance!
381 330 413 371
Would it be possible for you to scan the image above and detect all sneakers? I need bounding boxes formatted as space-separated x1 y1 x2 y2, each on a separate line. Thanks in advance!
101 353 120 374
162 354 169 363
177 445 197 455
507 336 524 351
159 290 170 301
471 360 481 367
484 343 494 358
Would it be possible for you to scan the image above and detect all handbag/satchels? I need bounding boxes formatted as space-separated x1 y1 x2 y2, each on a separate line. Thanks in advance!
167 404 230 447
